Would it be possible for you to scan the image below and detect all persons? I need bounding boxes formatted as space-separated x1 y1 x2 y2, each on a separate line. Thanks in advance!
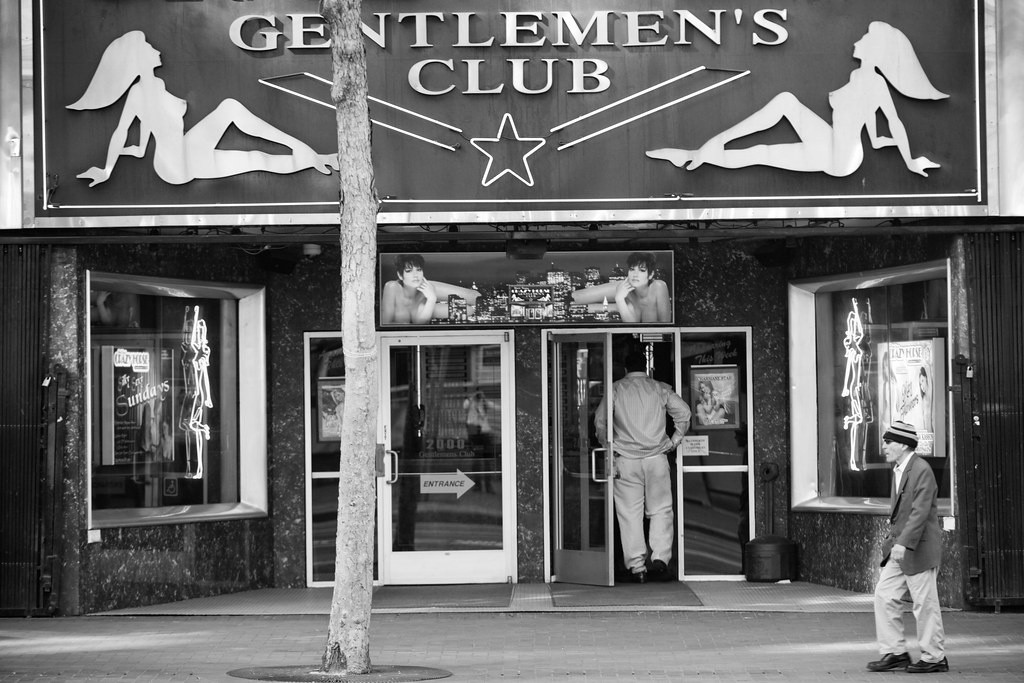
884 354 901 420
595 349 692 580
464 393 500 485
696 379 726 426
568 253 671 322
382 254 481 324
867 421 949 672
331 387 345 423
919 368 936 435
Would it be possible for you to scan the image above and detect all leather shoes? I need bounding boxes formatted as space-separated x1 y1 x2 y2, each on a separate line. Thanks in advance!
907 655 949 672
867 652 912 670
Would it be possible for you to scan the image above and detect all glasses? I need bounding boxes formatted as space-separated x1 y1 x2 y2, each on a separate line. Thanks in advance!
885 439 895 444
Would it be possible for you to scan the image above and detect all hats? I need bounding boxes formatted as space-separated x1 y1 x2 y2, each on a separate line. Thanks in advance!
883 421 918 449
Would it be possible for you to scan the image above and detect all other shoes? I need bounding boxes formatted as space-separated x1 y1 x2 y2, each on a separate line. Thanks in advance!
651 560 673 582
621 572 644 583
